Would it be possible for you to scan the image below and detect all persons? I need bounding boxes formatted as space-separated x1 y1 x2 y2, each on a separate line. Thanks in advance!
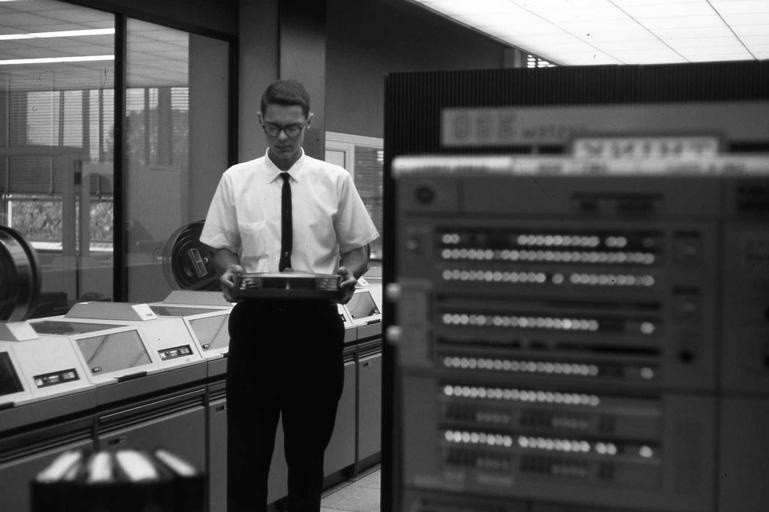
198 79 381 511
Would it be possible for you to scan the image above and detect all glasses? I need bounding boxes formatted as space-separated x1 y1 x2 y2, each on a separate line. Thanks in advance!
262 119 308 138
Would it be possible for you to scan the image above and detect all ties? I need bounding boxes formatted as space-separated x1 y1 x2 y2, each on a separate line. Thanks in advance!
278 172 294 274
